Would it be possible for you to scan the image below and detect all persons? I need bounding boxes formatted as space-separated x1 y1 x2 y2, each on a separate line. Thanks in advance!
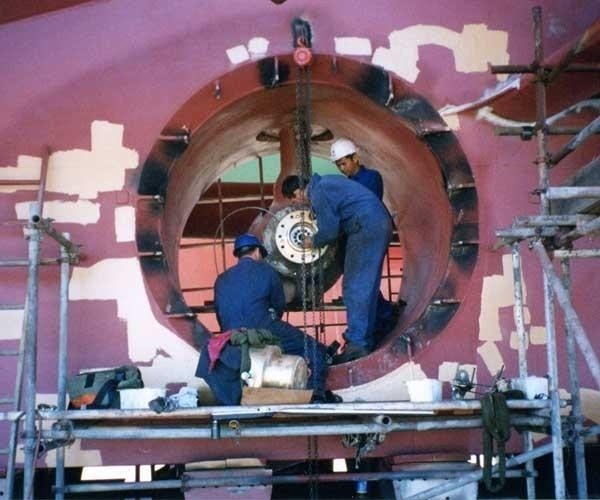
213 235 343 405
281 173 393 364
330 138 398 339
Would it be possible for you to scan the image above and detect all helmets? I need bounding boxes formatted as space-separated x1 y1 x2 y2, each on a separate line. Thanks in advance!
330 138 355 162
233 233 268 260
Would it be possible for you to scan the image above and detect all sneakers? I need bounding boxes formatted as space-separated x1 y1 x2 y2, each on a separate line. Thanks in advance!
334 346 371 366
311 391 342 404
